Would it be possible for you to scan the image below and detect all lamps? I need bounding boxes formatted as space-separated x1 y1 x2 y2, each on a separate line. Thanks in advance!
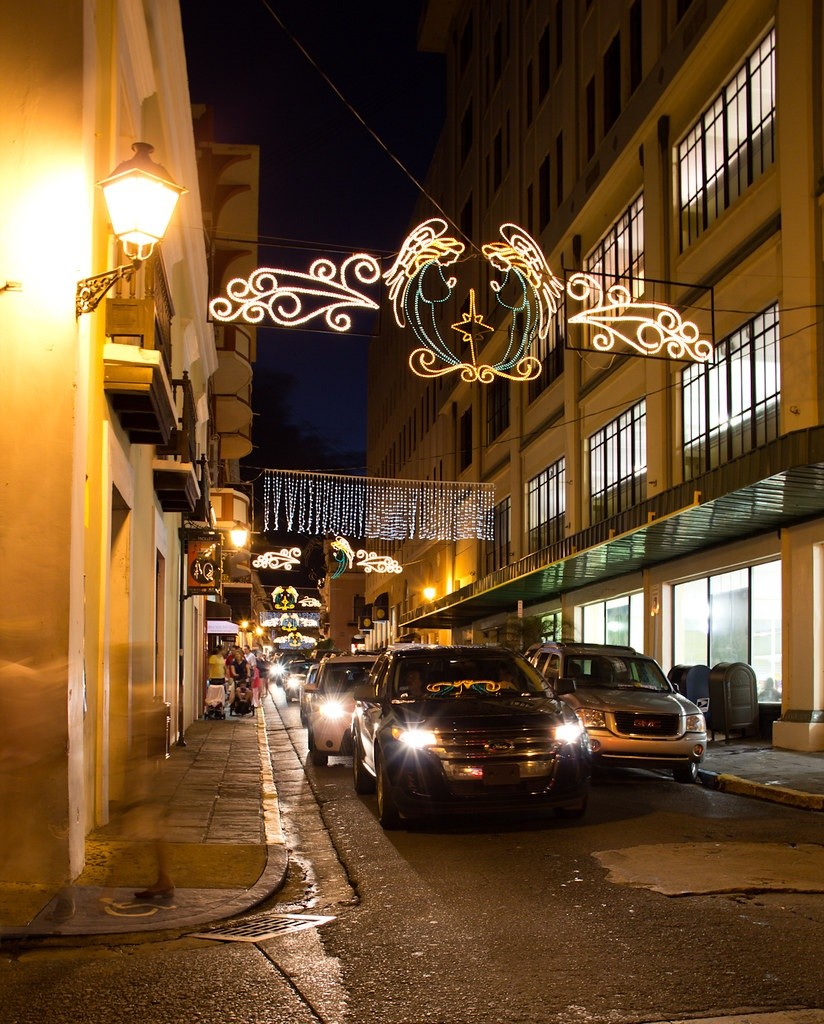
76 141 189 319
213 520 252 548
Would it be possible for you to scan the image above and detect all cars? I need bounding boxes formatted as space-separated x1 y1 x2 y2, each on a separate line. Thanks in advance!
299 659 328 725
268 652 304 686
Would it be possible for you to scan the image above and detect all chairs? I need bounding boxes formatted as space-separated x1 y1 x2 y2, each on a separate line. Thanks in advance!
401 664 436 697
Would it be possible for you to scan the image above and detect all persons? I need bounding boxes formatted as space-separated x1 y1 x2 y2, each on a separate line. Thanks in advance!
204 644 281 719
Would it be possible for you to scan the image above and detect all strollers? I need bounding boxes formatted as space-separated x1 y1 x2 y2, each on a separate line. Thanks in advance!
230 675 255 716
204 680 226 719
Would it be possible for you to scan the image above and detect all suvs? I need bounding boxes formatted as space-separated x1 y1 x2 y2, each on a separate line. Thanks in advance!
284 659 306 704
350 642 589 829
523 641 708 784
303 654 381 768
